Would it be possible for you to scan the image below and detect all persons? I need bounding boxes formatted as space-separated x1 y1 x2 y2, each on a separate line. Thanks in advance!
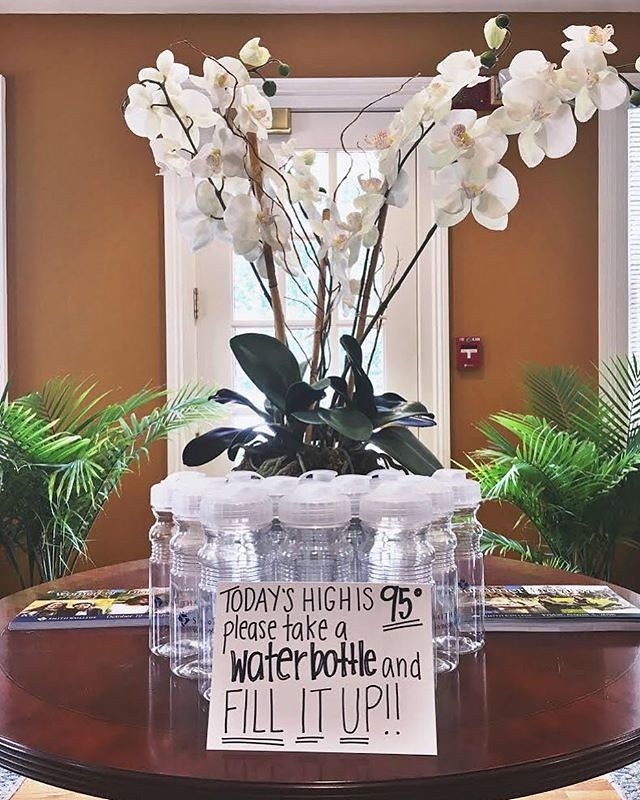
19 601 102 617
458 585 631 611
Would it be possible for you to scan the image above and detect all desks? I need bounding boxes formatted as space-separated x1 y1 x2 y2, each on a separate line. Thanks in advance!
0 555 640 799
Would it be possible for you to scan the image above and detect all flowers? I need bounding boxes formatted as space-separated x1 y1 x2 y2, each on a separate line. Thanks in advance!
121 14 640 479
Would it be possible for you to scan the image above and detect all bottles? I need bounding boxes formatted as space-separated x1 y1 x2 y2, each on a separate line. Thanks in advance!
148 470 485 703
